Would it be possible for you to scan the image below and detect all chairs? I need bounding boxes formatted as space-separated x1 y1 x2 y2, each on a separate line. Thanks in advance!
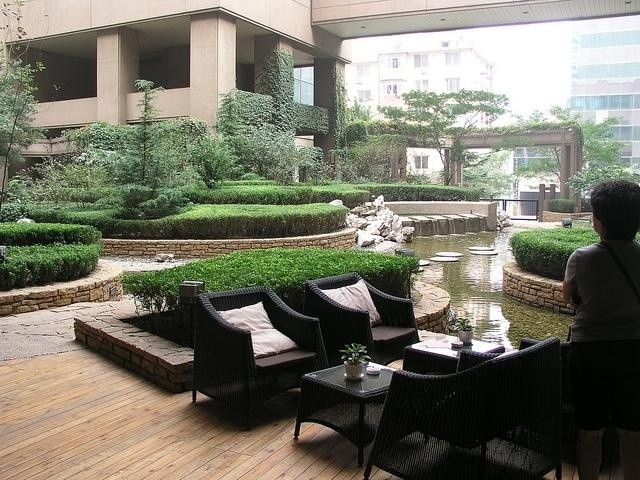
363 336 562 480
191 286 329 432
303 272 420 364
456 336 573 375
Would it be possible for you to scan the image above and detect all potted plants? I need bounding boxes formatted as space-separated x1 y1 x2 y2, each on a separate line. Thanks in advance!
339 342 371 382
453 318 473 346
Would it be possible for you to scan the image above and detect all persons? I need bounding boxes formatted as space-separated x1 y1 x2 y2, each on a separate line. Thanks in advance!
562 180 640 480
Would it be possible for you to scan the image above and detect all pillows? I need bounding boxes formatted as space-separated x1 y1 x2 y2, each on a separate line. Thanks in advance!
321 278 384 329
217 301 302 360
